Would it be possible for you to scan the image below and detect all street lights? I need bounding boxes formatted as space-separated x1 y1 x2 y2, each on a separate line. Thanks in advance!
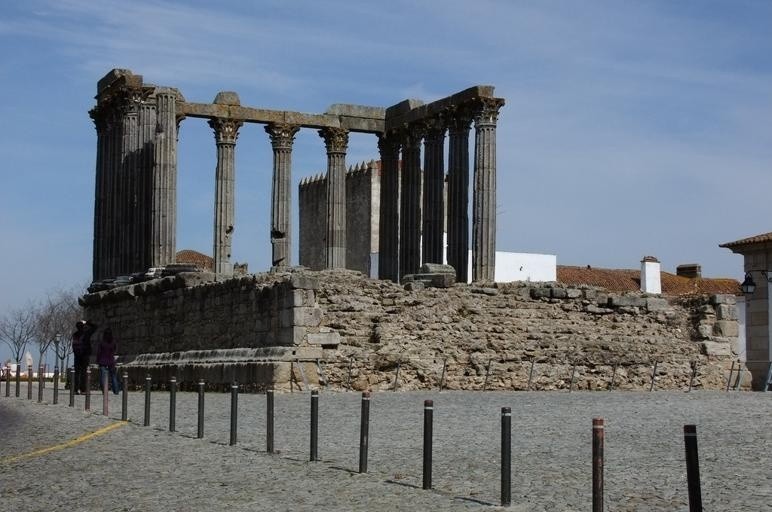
54 332 62 365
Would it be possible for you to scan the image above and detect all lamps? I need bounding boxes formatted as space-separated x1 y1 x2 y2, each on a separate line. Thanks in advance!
738 269 772 294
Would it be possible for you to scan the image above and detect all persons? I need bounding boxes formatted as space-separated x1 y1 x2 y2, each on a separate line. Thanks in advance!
70 318 96 393
97 330 121 395
